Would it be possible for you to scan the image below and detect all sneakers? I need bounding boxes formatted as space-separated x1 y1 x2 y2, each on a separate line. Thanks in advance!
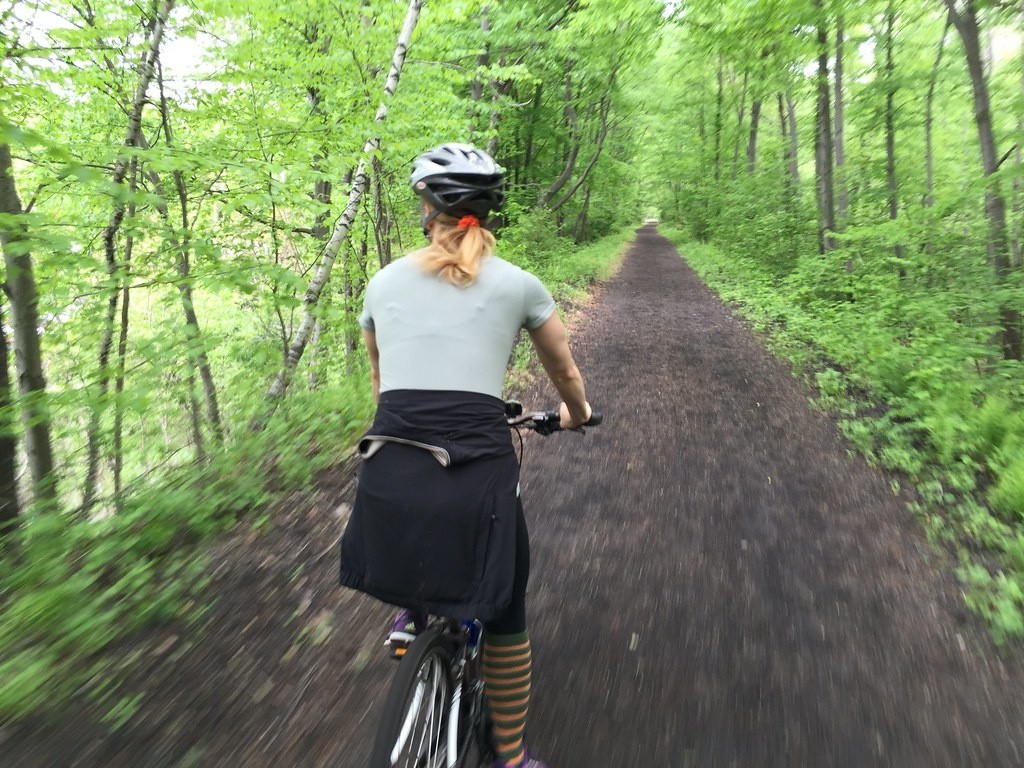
499 749 545 768
381 609 418 651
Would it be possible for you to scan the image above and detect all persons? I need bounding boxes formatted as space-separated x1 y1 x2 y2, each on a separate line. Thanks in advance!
339 142 591 768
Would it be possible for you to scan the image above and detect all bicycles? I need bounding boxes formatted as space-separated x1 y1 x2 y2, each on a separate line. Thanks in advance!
362 397 605 767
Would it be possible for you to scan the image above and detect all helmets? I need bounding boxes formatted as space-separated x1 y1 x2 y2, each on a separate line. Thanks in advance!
410 143 508 218
